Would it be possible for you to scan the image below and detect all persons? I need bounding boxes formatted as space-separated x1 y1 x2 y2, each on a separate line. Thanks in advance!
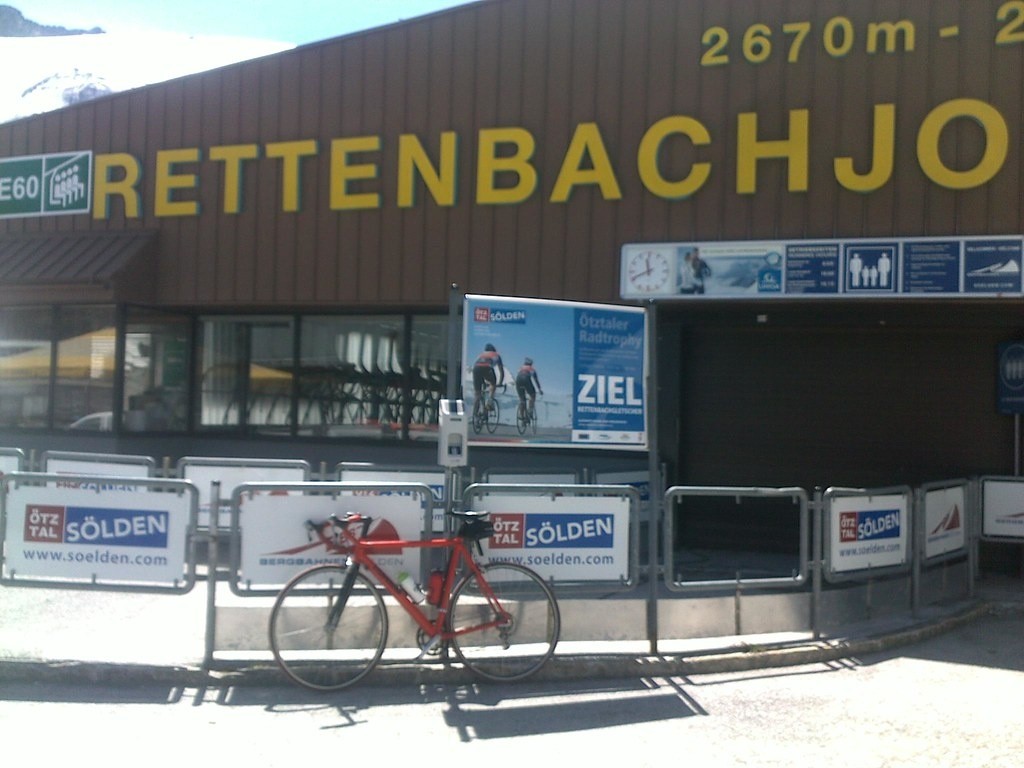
516 357 544 424
677 246 713 293
472 345 505 426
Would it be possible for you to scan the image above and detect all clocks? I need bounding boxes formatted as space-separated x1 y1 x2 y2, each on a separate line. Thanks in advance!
621 244 674 298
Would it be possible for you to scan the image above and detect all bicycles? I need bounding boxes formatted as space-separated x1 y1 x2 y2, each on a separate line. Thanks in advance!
469 383 507 435
267 511 563 693
514 390 545 436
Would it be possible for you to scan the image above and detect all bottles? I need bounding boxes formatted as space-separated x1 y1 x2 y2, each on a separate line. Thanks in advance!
427 569 443 605
398 572 425 604
